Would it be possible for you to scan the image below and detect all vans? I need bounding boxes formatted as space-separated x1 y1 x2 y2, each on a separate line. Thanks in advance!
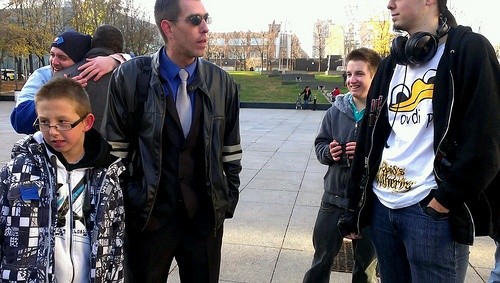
1 69 31 81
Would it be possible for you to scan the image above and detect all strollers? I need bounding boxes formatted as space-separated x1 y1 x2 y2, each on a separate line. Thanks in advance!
295 94 302 110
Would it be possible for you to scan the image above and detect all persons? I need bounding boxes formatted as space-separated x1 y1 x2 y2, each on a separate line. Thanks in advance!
10 32 133 134
106 0 243 283
296 70 347 110
336 0 500 283
302 48 383 283
51 25 123 139
0 79 126 283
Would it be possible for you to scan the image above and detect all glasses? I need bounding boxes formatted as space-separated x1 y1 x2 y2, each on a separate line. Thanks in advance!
168 13 209 26
33 113 89 132
51 30 92 65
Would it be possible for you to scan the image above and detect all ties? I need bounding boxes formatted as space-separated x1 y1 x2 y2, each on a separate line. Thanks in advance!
176 69 192 139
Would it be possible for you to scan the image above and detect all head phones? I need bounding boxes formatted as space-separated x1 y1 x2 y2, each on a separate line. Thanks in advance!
390 16 451 66
